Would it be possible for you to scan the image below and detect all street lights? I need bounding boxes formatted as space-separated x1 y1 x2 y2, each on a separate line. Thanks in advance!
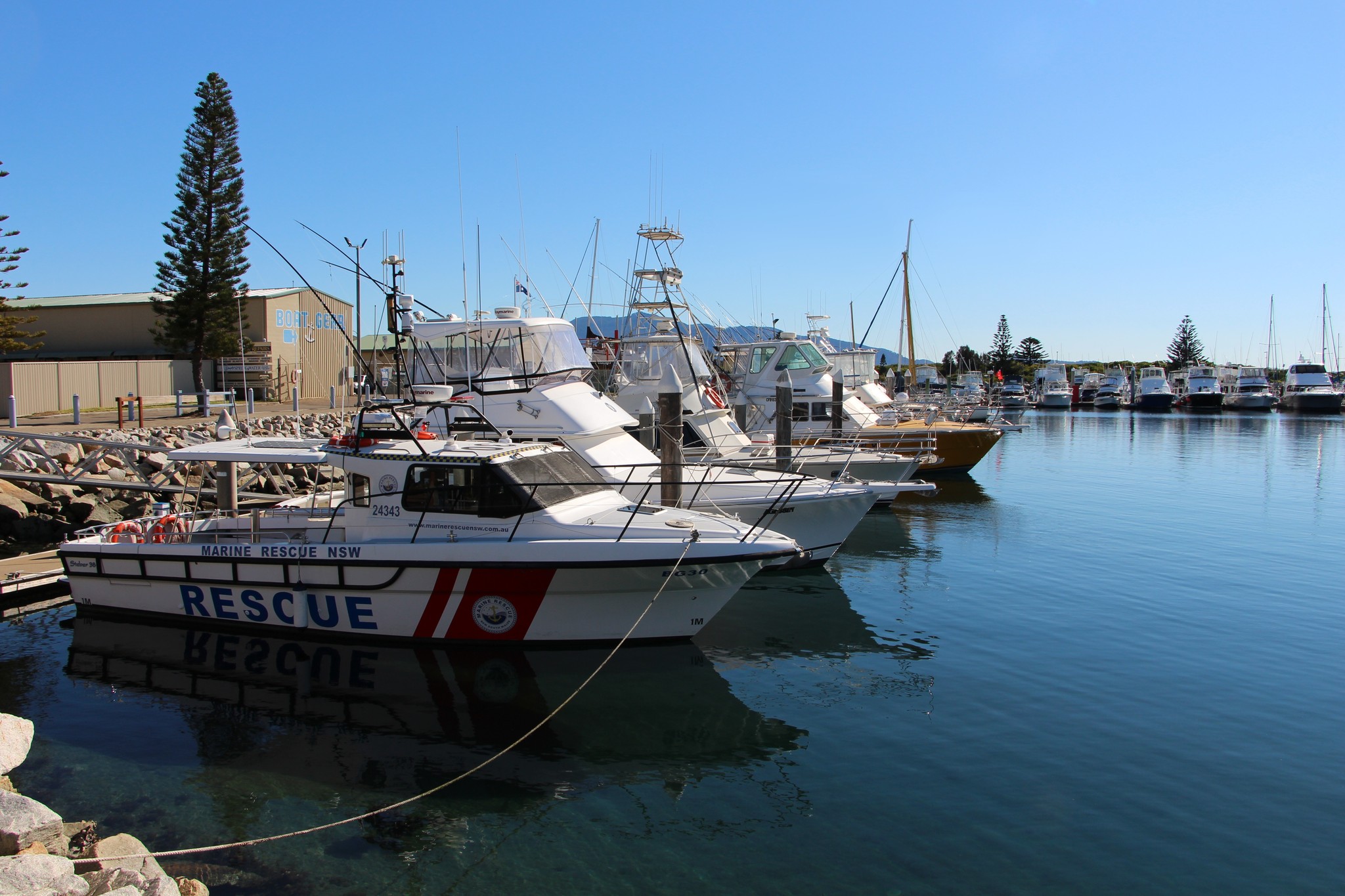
344 237 367 406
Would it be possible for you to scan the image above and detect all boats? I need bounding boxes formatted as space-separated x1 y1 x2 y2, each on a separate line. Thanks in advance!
1073 348 1227 411
1034 359 1074 408
1224 366 1277 411
55 153 1034 655
1278 362 1344 413
61 603 814 792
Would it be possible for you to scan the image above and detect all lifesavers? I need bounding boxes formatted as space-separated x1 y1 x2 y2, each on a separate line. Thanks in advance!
109 521 144 543
410 430 438 440
152 514 185 544
328 433 379 449
706 387 725 408
291 369 299 384
